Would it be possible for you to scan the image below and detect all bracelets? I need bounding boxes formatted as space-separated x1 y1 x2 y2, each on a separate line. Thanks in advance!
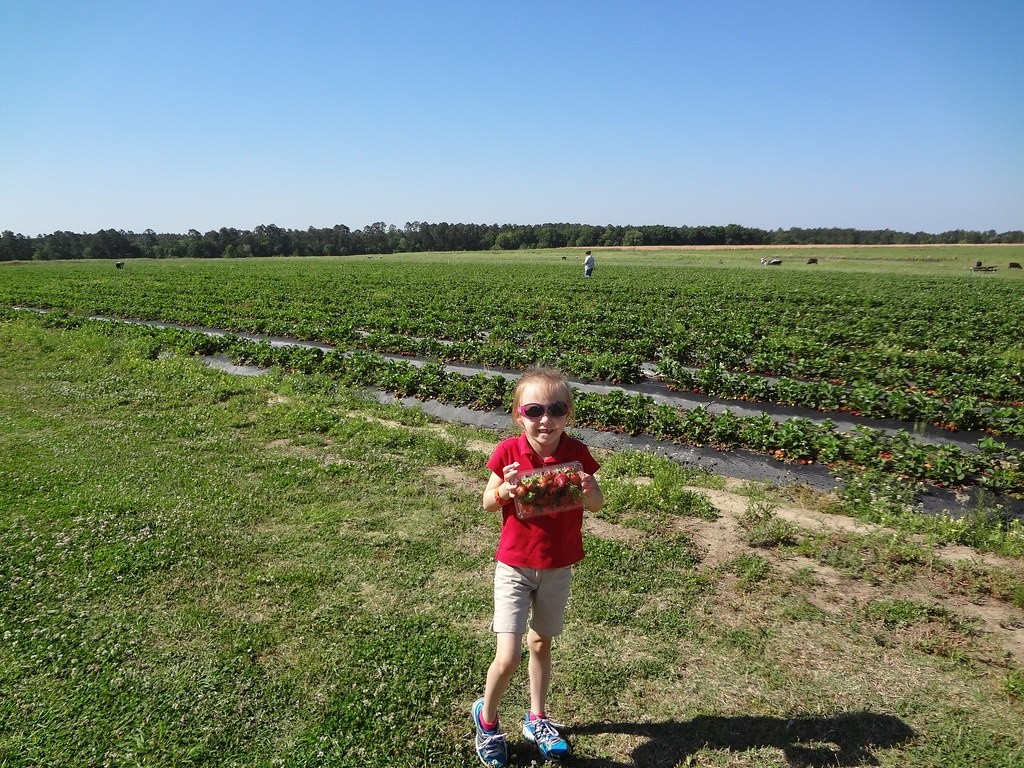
495 486 513 505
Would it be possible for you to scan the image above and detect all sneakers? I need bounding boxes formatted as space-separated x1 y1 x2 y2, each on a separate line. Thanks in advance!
471 697 508 768
522 710 572 762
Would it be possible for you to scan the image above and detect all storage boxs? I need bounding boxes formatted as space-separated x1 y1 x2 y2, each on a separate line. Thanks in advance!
510 460 589 520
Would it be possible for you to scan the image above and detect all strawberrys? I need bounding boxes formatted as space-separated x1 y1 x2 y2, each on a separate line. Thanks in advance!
513 466 583 515
594 374 1024 488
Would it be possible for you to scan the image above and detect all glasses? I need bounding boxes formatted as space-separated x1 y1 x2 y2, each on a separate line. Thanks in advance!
517 401 569 419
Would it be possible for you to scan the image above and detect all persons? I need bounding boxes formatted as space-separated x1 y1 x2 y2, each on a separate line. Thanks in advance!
472 369 605 768
581 251 596 276
